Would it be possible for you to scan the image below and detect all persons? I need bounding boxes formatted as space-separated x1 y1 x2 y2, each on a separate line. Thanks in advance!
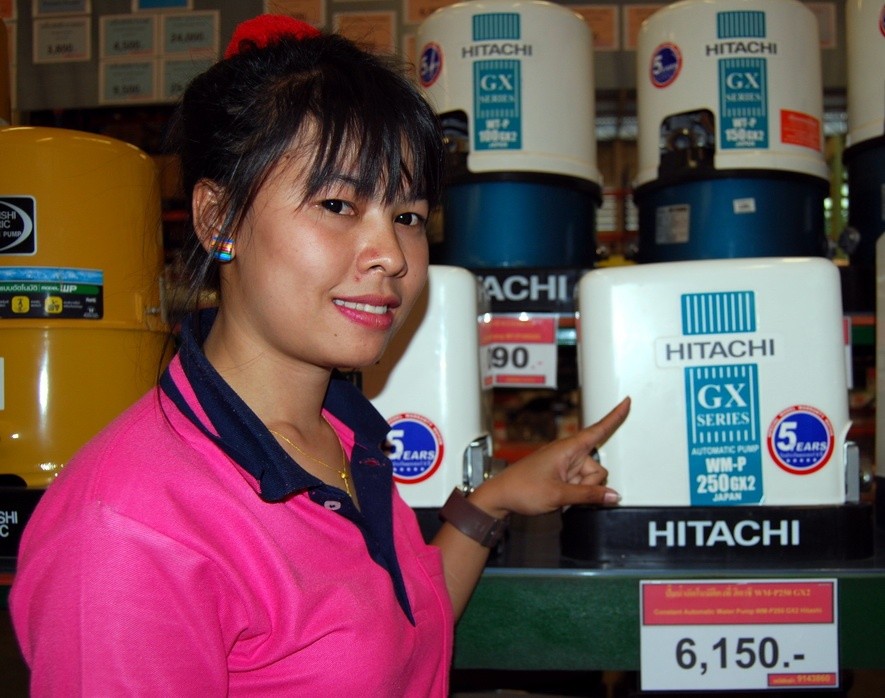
8 13 631 698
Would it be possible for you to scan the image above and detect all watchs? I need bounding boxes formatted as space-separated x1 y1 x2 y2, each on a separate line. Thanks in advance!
440 486 511 553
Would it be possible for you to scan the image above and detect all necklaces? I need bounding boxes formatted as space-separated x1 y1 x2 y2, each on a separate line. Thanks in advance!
267 413 353 498
